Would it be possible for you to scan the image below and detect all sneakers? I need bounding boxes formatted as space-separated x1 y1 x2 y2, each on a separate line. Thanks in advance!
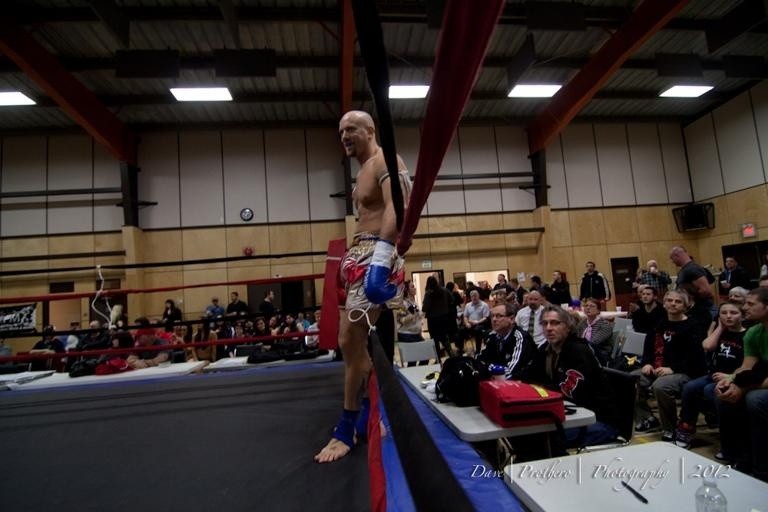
662 423 678 442
675 423 695 448
635 416 662 434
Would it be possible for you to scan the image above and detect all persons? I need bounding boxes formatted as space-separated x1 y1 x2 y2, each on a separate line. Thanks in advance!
313 106 416 465
30 244 768 485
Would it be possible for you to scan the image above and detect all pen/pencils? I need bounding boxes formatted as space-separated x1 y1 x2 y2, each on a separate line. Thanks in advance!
621 481 648 504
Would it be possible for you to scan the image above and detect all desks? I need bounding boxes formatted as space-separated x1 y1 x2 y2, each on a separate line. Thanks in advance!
398 362 598 460
573 309 629 321
200 348 335 370
6 358 203 393
0 369 56 386
495 434 767 512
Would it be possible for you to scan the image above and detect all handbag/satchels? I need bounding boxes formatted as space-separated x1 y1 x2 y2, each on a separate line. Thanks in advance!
435 356 490 406
479 379 566 429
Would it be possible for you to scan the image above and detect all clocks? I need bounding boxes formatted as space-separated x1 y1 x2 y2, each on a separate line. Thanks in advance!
240 208 255 222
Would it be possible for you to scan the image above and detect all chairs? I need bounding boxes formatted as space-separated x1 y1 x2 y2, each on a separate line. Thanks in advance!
394 335 438 365
234 343 265 356
610 315 635 329
610 329 652 361
566 365 642 452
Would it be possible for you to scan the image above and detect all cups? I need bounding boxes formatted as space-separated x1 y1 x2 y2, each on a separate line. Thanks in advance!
615 306 621 313
561 302 569 309
650 267 654 273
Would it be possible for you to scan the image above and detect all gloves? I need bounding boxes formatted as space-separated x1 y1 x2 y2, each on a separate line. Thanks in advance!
365 266 397 303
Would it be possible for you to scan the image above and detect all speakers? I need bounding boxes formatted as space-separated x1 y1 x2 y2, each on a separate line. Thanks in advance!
682 206 708 231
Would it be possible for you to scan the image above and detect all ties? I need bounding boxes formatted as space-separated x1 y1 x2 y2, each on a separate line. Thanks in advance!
528 311 534 337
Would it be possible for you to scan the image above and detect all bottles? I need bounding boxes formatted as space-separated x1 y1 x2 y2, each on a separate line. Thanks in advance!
488 365 506 381
696 478 728 511
304 327 314 346
